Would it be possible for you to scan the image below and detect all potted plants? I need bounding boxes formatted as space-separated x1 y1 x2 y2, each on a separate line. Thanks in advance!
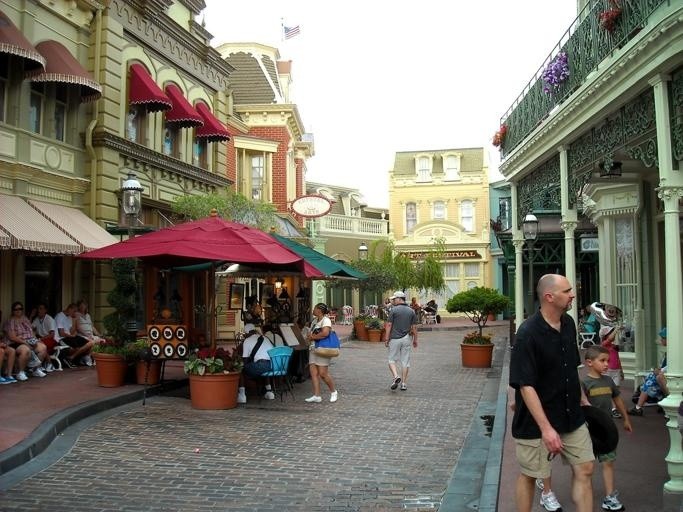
352 311 387 342
132 335 166 385
444 284 509 369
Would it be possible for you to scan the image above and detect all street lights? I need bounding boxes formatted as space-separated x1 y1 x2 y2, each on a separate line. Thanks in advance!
358 243 369 316
520 208 539 320
119 170 145 343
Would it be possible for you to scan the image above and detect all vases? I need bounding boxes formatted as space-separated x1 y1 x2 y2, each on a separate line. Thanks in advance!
188 372 239 410
90 351 128 388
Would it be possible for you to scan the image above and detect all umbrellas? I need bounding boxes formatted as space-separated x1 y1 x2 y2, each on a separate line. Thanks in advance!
79 207 327 350
170 229 368 309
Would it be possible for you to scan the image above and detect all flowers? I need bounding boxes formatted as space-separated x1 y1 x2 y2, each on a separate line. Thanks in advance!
91 337 131 358
181 346 242 376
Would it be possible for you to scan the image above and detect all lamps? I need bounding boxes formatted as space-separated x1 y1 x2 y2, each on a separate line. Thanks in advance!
242 275 312 327
597 157 622 180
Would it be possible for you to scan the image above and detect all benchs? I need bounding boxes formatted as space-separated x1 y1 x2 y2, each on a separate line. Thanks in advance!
42 345 69 371
578 330 596 350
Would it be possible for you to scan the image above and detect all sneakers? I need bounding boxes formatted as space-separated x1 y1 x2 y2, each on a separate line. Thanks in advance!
391 376 401 390
305 396 322 403
611 409 623 418
401 382 407 390
330 390 338 402
0 364 54 384
63 357 77 368
601 495 625 511
627 405 644 416
265 391 275 400
237 393 246 404
535 478 562 512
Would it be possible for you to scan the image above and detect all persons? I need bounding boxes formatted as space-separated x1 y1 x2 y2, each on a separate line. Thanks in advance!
597 323 625 418
409 297 424 321
384 298 393 309
581 347 635 511
235 323 274 403
384 291 417 390
578 308 588 323
627 327 666 415
327 308 339 321
424 300 437 314
509 273 593 511
303 303 340 403
289 318 312 382
578 304 599 343
535 475 564 511
0 297 105 383
261 324 285 350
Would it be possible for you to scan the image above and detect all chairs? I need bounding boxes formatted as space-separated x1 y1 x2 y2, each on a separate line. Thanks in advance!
259 345 299 403
327 299 441 325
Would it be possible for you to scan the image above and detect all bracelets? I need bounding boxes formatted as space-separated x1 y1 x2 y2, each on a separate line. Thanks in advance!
385 339 388 341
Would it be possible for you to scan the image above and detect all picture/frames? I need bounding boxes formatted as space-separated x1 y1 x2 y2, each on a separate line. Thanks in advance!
228 282 244 310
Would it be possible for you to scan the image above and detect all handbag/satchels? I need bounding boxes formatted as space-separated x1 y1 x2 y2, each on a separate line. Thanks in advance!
314 329 340 357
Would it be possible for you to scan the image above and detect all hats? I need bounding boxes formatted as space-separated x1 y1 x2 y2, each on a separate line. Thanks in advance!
388 291 406 300
244 323 255 334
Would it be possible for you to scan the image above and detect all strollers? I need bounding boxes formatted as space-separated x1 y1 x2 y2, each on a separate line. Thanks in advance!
632 351 667 416
